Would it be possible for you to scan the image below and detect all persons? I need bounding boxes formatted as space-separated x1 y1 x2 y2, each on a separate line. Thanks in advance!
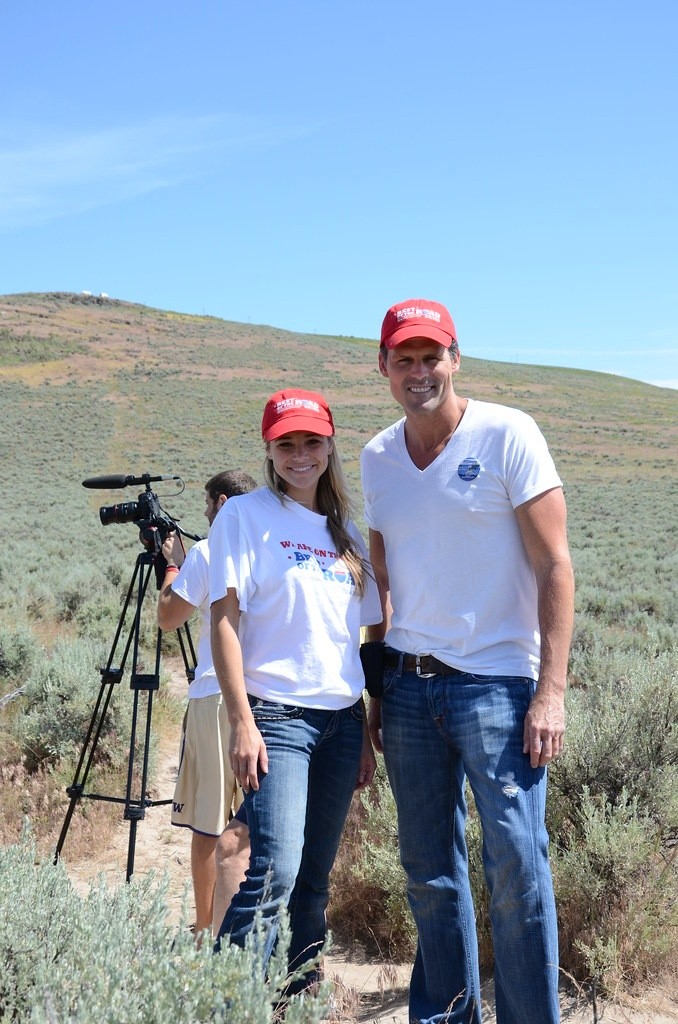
353 299 579 1023
203 387 386 1017
158 466 260 944
208 791 256 943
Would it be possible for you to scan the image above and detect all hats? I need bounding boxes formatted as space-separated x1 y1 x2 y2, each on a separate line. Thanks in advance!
261 388 333 442
379 300 458 350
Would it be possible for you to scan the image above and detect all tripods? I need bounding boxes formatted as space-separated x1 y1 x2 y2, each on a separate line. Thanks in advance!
54 554 199 883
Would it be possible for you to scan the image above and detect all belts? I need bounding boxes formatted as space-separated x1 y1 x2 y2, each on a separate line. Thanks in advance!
383 652 462 678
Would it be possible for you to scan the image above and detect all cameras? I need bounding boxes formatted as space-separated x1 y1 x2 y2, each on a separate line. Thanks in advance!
100 492 176 553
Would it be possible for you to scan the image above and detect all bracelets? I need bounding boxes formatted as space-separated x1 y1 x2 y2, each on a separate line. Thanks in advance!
166 563 182 568
163 567 180 573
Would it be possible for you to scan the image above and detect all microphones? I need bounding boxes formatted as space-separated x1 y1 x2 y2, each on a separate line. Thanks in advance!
82 473 181 489
